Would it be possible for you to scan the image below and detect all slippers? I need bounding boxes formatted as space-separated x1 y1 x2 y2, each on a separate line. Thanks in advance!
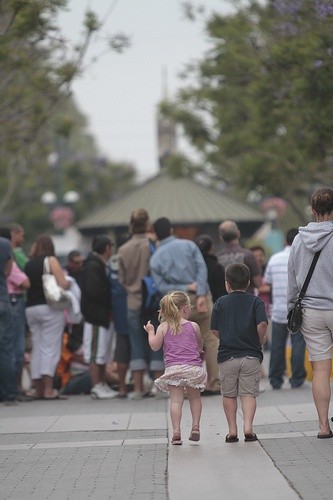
41 389 68 400
317 429 333 438
226 434 239 442
245 433 257 442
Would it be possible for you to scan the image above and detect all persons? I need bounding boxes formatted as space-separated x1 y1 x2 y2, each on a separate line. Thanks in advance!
287 193 333 438
144 291 206 445
209 263 268 442
0 205 307 402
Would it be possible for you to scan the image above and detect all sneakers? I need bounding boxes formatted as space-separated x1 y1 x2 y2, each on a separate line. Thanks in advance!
5 393 32 402
91 382 120 399
127 390 144 400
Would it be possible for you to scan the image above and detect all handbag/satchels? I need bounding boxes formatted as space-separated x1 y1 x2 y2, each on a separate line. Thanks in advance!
142 240 161 312
42 256 71 309
287 302 302 333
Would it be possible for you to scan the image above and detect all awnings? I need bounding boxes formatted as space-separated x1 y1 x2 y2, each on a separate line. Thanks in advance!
74 171 266 230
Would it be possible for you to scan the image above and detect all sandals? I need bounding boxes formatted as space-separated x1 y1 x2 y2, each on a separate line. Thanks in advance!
189 425 200 441
171 432 182 445
